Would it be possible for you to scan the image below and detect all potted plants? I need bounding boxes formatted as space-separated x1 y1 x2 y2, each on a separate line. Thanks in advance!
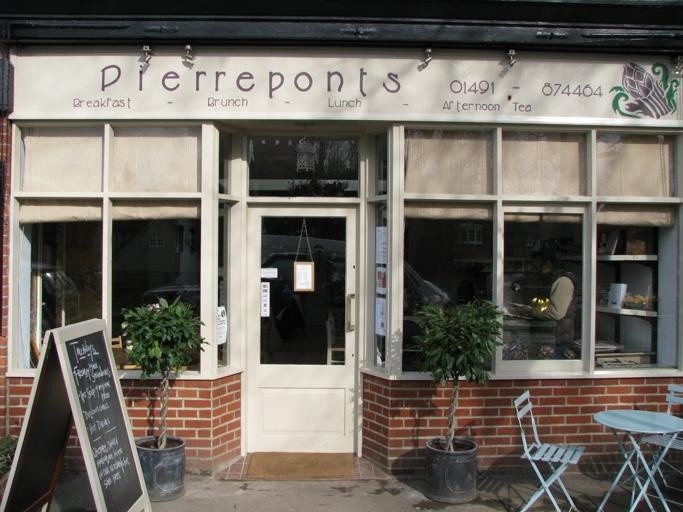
396 292 508 504
110 292 211 505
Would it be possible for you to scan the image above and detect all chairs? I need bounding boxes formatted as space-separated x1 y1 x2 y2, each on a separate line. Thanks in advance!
635 382 683 508
322 311 346 366
509 388 587 512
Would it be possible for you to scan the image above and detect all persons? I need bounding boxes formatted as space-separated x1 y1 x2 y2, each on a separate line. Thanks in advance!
511 251 554 312
515 248 575 358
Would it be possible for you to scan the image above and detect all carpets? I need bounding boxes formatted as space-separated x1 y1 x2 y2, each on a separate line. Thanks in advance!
240 448 362 483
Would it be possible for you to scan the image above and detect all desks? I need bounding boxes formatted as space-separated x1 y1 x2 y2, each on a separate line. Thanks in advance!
591 409 683 511
394 305 559 358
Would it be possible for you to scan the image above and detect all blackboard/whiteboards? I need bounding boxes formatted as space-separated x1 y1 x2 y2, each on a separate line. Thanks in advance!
53 318 149 512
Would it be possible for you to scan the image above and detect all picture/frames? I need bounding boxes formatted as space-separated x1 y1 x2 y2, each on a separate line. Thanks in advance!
292 261 315 293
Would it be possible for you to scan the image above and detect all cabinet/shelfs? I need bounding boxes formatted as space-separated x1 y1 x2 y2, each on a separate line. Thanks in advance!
449 244 661 321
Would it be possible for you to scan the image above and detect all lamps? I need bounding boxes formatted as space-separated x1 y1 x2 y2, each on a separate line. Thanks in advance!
421 48 433 64
183 44 195 67
504 46 519 69
134 44 152 73
